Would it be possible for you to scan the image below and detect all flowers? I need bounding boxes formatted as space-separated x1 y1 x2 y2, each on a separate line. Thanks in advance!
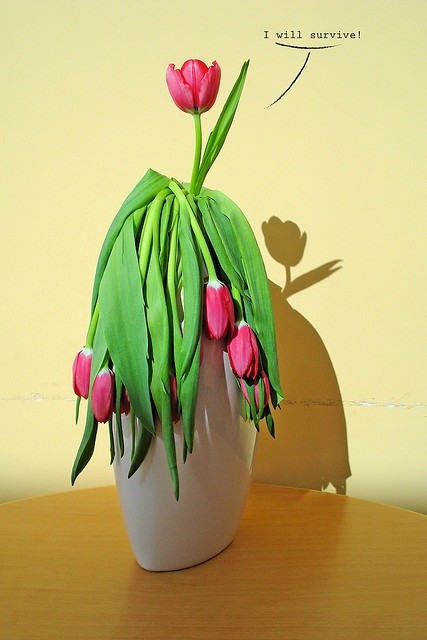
72 59 286 503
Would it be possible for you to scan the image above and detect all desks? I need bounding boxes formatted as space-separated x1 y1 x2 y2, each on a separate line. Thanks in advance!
0 484 427 639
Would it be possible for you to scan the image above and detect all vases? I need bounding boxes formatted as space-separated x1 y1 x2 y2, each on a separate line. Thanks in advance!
112 286 258 573
